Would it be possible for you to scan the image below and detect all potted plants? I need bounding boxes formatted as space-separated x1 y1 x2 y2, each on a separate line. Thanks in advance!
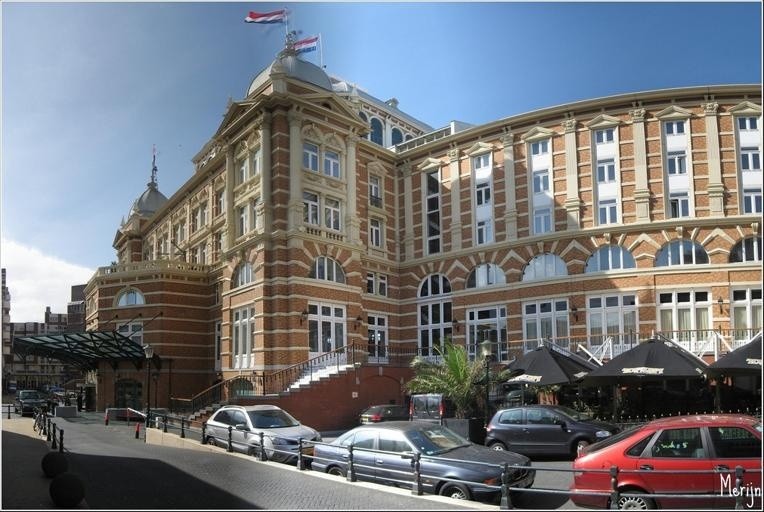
401 340 525 442
452 317 459 332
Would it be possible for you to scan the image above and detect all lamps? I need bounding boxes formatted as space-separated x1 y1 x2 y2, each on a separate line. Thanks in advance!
354 314 363 330
571 304 579 321
717 296 724 314
300 308 308 326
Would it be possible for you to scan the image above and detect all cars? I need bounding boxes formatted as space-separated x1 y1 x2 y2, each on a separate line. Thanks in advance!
568 414 762 510
310 420 536 502
205 402 323 464
484 405 621 459
359 404 409 426
42 384 85 399
507 391 538 405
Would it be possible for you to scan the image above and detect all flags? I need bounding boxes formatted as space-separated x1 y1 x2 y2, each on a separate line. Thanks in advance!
244 7 286 24
291 34 319 55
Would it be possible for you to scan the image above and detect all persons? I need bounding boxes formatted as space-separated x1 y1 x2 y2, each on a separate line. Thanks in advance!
76 393 82 412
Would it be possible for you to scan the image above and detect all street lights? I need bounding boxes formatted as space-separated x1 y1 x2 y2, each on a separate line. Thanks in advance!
153 372 160 408
217 372 223 401
143 343 156 426
476 339 496 428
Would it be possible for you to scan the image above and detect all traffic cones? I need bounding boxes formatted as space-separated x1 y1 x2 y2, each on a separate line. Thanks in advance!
105 415 109 424
136 422 140 439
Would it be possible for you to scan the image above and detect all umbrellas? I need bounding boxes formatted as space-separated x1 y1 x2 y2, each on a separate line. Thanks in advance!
493 329 762 387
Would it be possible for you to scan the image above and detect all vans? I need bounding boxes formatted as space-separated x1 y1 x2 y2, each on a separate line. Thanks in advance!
409 391 499 443
8 381 17 392
106 408 167 423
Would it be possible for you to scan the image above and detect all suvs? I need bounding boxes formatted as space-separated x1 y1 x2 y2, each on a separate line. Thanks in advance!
14 390 48 416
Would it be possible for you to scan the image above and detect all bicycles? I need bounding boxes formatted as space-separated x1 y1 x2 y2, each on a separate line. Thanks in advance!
32 407 53 435
